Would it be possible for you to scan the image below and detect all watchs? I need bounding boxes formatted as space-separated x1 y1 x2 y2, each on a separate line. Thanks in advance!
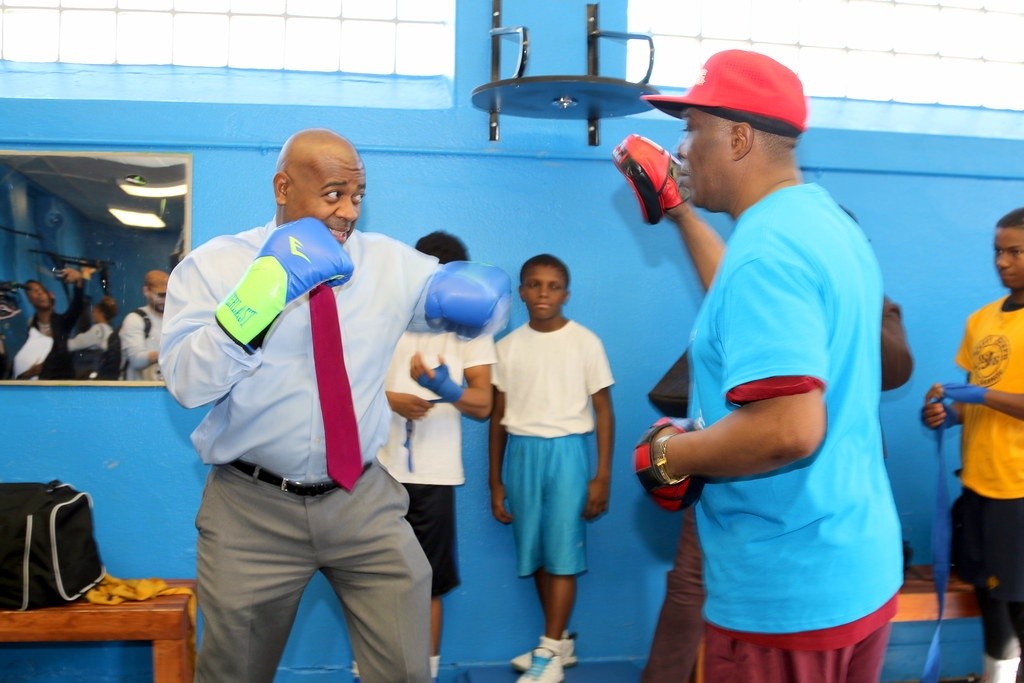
652 433 690 485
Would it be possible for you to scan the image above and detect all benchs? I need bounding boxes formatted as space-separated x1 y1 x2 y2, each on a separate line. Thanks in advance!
0 579 197 683
697 565 981 683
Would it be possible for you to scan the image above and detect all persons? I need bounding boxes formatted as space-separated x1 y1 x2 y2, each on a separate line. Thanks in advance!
485 252 617 682
350 231 495 683
918 206 1024 683
157 125 511 683
1 269 170 384
609 49 907 683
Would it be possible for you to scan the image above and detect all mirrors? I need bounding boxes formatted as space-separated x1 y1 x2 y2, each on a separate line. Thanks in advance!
0 150 195 391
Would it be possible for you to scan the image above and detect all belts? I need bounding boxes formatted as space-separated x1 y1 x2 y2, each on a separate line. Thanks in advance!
230 458 373 497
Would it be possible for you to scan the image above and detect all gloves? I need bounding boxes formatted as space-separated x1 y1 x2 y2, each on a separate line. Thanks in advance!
424 260 511 340
215 217 353 354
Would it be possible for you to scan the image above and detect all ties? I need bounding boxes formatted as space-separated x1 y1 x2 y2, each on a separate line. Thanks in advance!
309 282 364 492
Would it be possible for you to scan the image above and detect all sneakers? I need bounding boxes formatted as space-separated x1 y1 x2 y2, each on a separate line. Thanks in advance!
516 647 565 683
510 632 580 672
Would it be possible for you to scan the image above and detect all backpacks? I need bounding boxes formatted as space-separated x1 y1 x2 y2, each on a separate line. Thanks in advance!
101 308 151 381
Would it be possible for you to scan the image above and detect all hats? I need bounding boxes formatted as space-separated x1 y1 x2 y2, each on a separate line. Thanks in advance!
640 49 806 136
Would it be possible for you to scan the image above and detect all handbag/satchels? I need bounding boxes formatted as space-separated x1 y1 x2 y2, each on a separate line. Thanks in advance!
0 479 107 612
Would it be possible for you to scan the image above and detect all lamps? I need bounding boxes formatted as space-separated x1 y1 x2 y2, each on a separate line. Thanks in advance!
115 178 189 199
104 203 167 232
